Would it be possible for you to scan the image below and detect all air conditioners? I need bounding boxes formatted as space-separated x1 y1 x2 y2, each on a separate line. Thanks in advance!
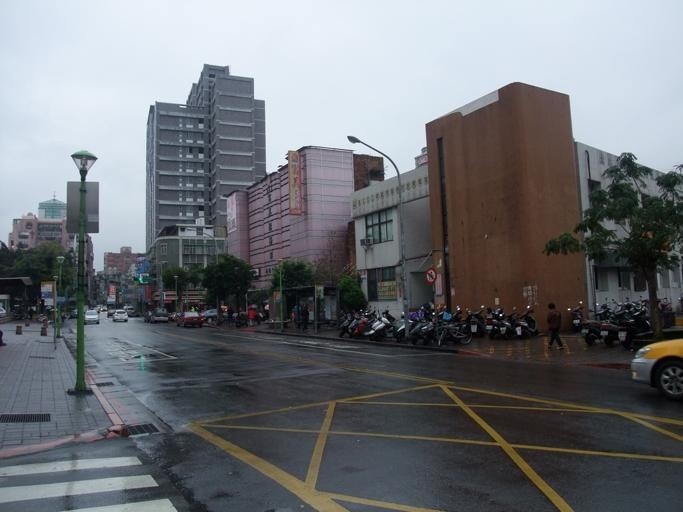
359 238 373 245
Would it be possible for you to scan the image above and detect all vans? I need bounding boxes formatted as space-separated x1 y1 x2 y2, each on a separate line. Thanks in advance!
123 305 136 316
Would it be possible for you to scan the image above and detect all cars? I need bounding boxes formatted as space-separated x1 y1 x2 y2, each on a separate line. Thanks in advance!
107 308 115 317
630 338 682 401
83 309 98 325
176 311 203 328
112 310 128 322
143 307 266 323
69 307 84 318
92 304 107 313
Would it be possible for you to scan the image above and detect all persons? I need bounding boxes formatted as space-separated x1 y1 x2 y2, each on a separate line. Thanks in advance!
218 305 256 326
545 302 566 350
301 306 310 332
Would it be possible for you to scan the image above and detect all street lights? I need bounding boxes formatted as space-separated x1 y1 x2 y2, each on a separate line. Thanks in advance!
172 276 179 321
52 276 58 328
55 256 65 338
186 228 221 326
275 258 283 331
346 135 409 342
66 151 97 394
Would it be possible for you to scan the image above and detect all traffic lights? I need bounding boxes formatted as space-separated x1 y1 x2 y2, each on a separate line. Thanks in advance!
134 277 142 282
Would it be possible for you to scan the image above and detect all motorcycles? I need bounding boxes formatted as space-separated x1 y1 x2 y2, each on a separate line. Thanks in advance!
235 313 248 329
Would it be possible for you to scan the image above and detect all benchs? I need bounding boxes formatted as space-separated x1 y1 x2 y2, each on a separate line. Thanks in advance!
264 317 331 334
